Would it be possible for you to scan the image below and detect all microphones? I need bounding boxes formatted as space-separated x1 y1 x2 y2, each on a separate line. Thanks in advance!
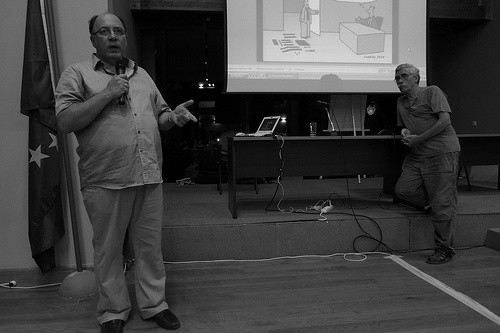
316 100 330 107
115 59 127 105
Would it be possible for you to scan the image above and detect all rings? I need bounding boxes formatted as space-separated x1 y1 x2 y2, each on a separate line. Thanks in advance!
406 138 410 142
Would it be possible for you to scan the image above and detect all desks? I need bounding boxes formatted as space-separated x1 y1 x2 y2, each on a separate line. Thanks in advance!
227 132 500 218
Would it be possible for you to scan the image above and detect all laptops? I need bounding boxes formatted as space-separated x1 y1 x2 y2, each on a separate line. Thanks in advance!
245 116 281 136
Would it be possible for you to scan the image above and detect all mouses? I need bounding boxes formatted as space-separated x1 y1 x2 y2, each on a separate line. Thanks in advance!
235 132 246 136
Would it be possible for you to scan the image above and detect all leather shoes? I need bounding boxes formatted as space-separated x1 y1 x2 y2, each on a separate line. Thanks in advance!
426 248 451 264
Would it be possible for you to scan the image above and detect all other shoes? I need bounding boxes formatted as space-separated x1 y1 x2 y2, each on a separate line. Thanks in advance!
144 309 180 329
101 319 127 333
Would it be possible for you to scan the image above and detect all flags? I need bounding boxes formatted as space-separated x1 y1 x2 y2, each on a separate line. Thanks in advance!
20 0 65 274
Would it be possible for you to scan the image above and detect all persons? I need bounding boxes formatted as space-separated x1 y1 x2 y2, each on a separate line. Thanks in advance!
392 63 461 261
54 13 199 333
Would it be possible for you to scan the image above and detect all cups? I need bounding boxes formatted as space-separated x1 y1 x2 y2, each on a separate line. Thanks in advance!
310 122 317 136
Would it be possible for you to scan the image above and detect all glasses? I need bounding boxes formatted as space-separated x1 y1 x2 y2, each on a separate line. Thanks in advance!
394 73 412 81
92 27 125 36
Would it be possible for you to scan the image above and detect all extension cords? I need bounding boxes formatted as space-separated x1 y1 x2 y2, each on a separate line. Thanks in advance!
321 205 334 213
